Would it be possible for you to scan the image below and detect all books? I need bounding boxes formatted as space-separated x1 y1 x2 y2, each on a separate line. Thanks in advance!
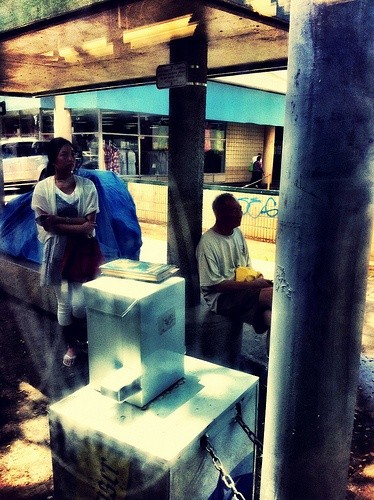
98 258 179 283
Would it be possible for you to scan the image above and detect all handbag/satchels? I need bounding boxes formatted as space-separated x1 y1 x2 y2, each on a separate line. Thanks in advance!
248 163 254 172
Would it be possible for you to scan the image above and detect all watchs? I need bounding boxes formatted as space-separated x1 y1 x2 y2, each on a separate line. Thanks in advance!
66 217 70 224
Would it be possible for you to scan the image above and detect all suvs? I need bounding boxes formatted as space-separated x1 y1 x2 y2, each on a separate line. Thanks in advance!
0 137 51 194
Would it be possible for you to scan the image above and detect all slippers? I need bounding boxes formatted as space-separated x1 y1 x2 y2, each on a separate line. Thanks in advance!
63 348 77 368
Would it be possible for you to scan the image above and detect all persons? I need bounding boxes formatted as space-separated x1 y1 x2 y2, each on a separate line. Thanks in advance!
195 192 273 351
251 157 266 189
249 153 263 186
31 137 103 367
